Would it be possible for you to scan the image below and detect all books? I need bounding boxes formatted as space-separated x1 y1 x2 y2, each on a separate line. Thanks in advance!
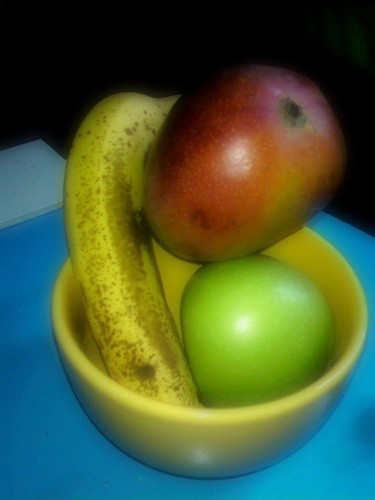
0 139 67 229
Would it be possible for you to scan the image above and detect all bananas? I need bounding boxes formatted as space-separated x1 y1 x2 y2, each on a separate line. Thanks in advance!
62 90 200 411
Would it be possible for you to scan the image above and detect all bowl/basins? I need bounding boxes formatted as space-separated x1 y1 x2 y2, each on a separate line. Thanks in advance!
50 223 368 479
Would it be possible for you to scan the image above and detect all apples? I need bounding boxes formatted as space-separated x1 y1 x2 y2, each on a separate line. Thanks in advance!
181 254 333 409
141 61 344 267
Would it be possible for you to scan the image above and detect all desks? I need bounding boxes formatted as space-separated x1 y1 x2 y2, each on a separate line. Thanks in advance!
0 208 374 495
